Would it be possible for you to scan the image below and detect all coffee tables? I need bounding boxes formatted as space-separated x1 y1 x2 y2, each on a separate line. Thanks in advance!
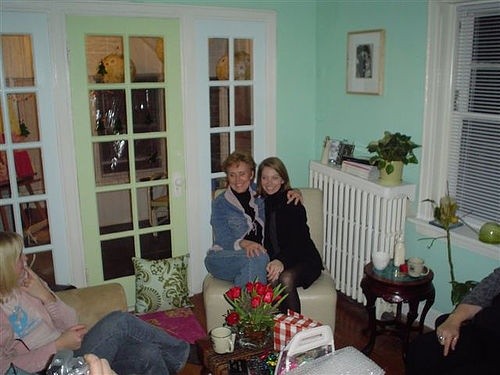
195 334 274 375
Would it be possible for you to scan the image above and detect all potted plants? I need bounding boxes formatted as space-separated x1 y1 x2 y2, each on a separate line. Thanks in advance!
368 129 423 186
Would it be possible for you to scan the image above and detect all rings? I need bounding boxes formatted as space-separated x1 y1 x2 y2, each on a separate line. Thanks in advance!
454 338 457 340
442 337 446 341
439 336 442 340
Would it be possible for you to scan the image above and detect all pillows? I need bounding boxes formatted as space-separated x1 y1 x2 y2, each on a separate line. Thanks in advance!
139 307 207 345
132 253 196 316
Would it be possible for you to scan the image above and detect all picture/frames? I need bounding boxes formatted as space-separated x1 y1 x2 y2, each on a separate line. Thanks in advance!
346 29 388 96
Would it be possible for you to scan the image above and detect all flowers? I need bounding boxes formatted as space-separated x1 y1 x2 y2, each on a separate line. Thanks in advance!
222 274 290 332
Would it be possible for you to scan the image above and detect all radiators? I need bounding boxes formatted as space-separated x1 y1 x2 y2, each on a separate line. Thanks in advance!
307 160 416 307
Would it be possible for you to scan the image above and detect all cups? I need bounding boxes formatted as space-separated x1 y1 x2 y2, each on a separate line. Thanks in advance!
210 327 234 354
408 256 430 277
372 251 390 270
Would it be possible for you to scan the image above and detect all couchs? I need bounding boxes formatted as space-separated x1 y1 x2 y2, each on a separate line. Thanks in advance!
202 187 337 339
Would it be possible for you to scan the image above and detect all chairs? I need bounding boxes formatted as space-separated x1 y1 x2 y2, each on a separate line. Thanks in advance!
148 172 168 237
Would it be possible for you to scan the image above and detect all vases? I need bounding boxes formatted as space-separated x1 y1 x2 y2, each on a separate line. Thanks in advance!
243 326 268 346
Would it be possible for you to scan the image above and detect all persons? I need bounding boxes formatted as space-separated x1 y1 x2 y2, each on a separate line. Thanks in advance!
0 230 204 375
204 150 304 288
83 354 118 375
416 267 500 375
253 156 325 317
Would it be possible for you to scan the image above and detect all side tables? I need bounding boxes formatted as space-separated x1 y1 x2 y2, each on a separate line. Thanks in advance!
360 260 435 356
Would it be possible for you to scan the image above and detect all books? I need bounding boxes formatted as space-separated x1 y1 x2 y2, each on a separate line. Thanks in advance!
342 161 380 180
